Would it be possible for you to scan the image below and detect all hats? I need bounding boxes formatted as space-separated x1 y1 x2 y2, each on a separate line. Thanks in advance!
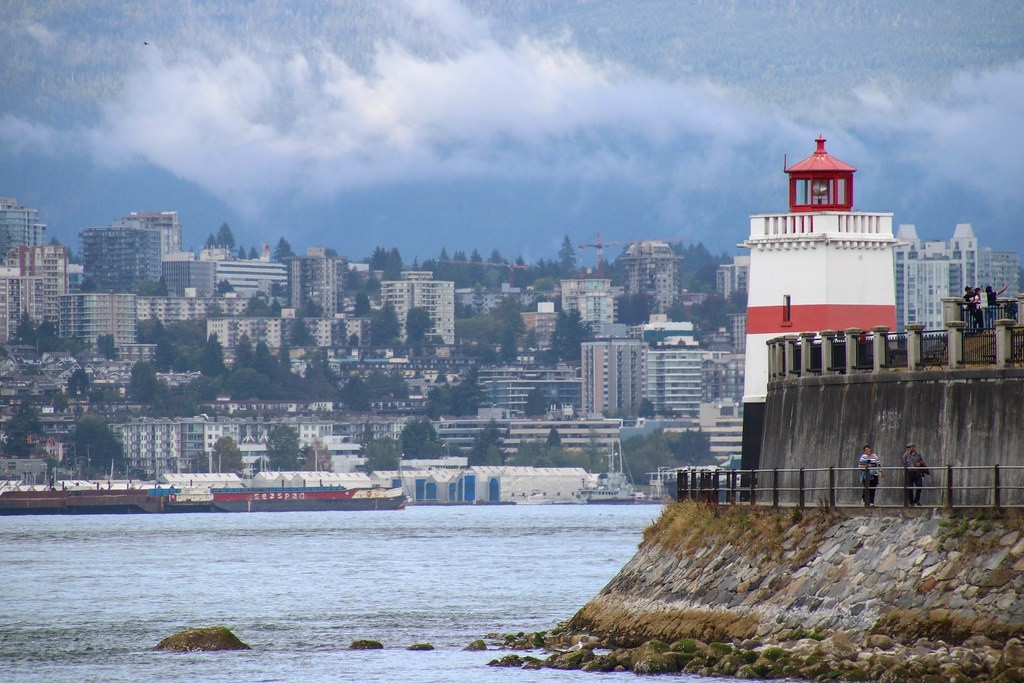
965 285 972 291
905 443 915 447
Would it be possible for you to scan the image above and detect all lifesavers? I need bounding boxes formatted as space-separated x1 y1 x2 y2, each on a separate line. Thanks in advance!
171 494 176 501
367 491 371 497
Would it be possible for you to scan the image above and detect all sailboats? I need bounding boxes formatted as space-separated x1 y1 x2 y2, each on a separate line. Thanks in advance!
575 437 639 505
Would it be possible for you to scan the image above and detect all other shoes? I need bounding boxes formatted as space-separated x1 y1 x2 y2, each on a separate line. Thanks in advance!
861 499 865 505
914 499 921 506
870 503 874 507
910 500 914 506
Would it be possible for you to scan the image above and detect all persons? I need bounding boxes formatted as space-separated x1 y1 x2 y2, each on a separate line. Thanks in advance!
963 282 1009 330
902 444 928 506
859 445 882 506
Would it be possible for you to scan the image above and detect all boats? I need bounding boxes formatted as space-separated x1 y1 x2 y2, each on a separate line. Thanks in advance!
208 480 413 513
